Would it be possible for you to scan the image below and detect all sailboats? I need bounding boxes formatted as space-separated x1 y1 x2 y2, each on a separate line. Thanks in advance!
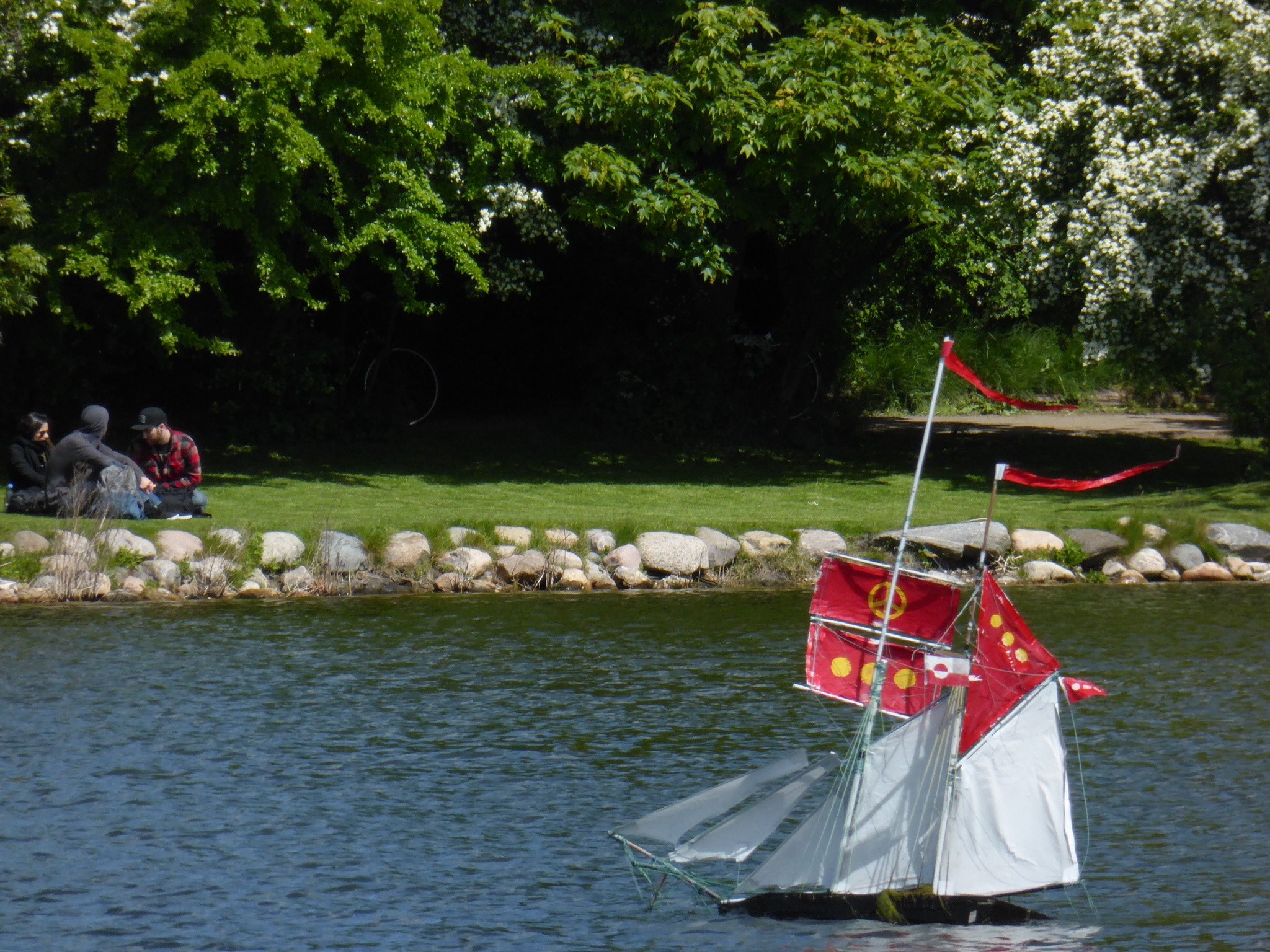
608 330 1085 917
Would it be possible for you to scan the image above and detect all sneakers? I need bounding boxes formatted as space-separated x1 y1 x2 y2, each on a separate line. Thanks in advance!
156 502 192 520
143 504 178 520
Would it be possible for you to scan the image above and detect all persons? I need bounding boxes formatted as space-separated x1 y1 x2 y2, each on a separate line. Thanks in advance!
126 406 204 517
5 411 61 515
41 404 195 521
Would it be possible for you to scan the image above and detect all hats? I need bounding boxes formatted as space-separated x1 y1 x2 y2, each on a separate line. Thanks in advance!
133 406 168 432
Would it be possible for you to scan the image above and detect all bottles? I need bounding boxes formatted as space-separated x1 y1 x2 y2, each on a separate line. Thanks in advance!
5 484 16 506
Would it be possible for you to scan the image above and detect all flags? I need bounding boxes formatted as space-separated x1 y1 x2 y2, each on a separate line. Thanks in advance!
808 555 1108 761
